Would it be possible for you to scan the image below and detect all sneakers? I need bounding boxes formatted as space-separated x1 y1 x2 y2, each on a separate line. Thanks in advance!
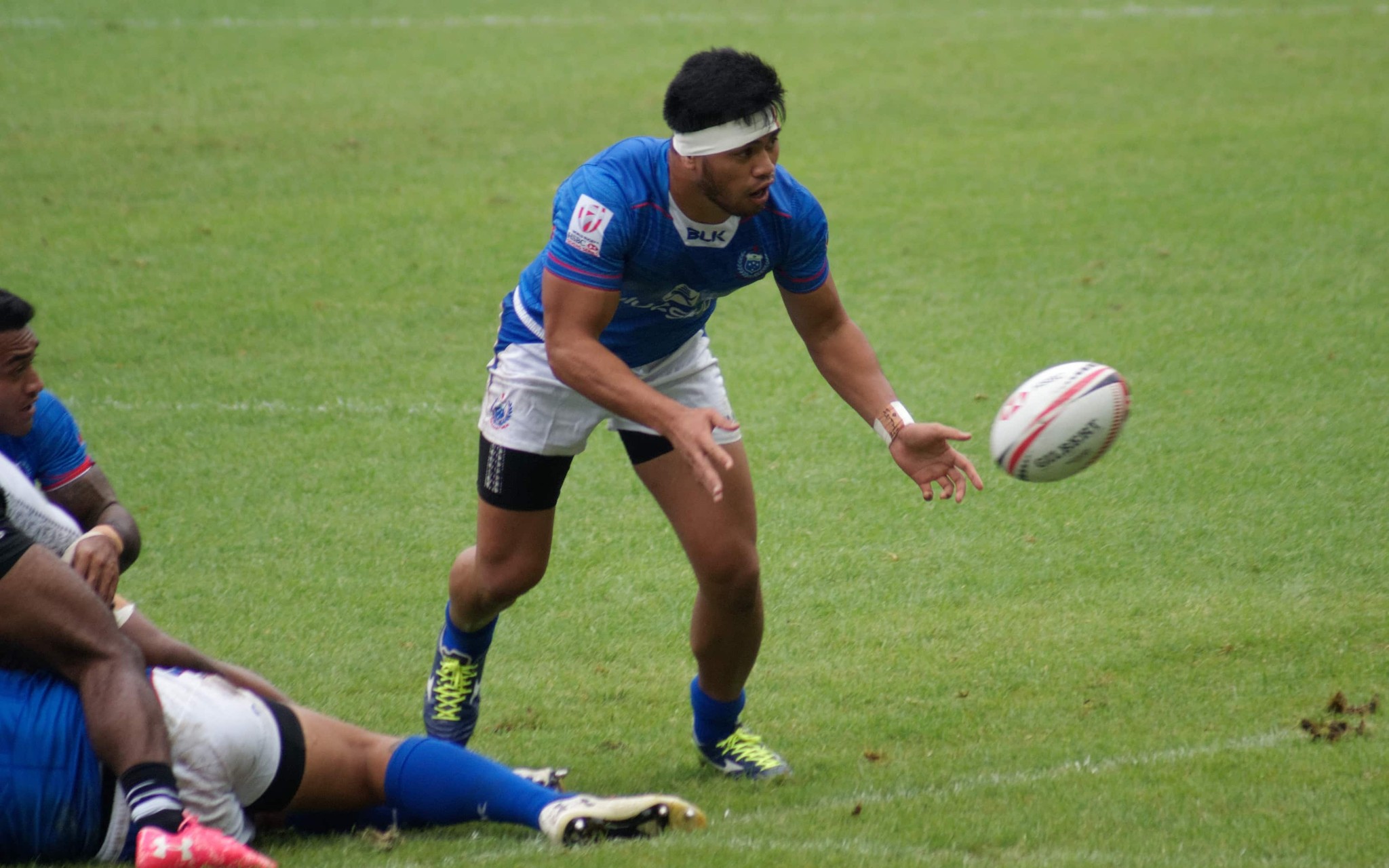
536 793 707 848
136 808 279 868
421 621 495 749
692 727 790 782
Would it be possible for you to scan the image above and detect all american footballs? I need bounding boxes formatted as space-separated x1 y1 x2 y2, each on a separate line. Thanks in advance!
989 362 1131 483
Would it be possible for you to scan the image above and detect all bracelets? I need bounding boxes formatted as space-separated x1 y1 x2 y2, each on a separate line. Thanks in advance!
90 523 124 555
873 401 915 450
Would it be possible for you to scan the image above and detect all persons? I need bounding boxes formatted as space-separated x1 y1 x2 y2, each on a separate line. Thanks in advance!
423 46 982 787
0 275 706 868
